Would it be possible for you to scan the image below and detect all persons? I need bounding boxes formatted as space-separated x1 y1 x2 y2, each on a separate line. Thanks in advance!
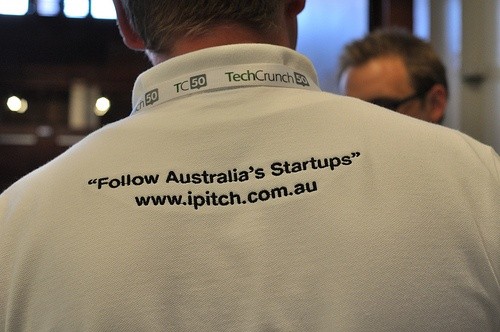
0 0 499 331
336 25 448 127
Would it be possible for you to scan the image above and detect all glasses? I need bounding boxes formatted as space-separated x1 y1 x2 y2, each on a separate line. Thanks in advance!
367 93 420 112
